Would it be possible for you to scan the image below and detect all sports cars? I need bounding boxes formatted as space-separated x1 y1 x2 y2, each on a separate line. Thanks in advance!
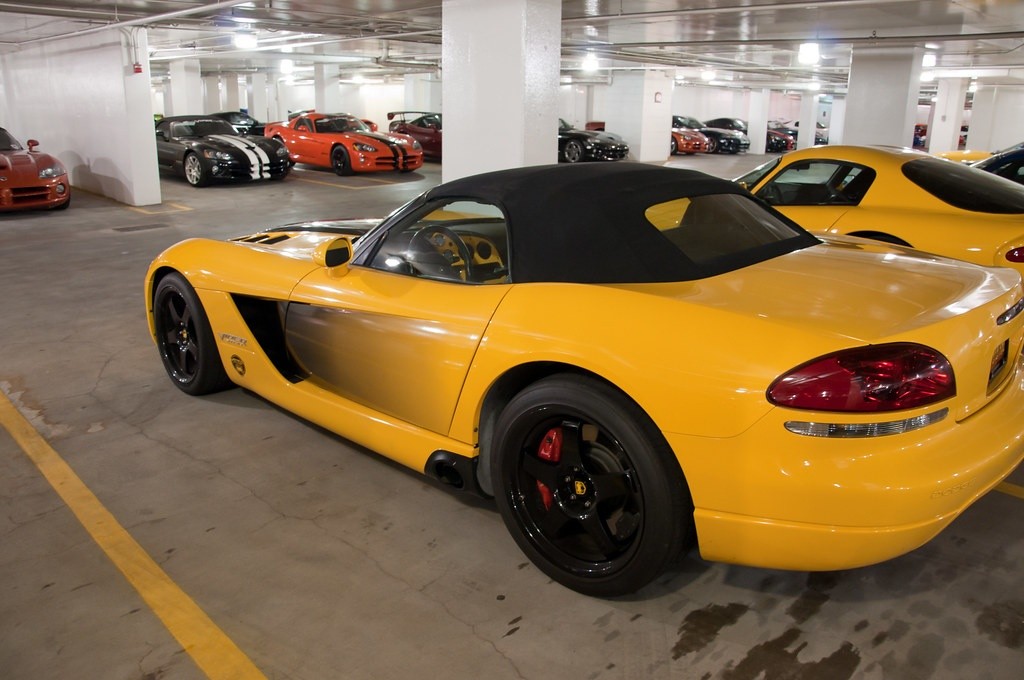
155 114 291 188
558 118 630 165
0 125 73 212
140 161 1024 599
717 143 1024 284
264 112 425 176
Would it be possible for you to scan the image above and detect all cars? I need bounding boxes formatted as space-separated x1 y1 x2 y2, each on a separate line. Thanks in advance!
912 122 1024 184
704 118 830 152
686 116 750 154
388 111 443 160
209 111 272 138
584 116 709 157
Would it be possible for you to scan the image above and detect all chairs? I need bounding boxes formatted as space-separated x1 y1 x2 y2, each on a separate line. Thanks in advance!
336 119 350 133
